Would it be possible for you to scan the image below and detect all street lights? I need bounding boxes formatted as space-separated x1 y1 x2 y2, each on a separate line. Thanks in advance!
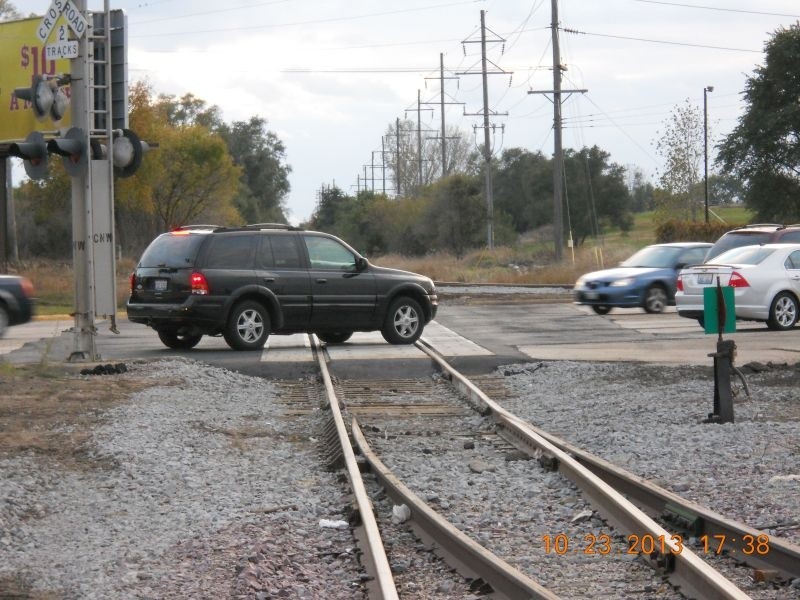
703 85 714 223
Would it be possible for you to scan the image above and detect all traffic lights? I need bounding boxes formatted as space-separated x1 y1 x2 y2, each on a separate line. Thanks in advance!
113 129 152 177
47 127 88 176
7 131 50 178
14 73 54 121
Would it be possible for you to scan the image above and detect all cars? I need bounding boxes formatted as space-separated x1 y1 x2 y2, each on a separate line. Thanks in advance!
674 243 800 331
0 275 38 338
572 242 716 316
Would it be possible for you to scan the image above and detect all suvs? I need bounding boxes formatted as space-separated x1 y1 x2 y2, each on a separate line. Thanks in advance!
126 224 439 352
703 223 800 265
